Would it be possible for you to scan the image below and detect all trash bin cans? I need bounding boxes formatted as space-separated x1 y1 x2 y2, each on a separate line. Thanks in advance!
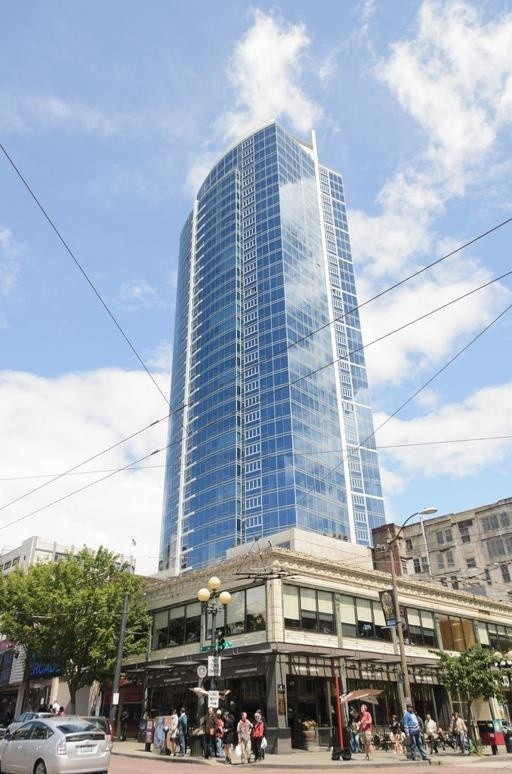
189 734 202 757
477 720 506 756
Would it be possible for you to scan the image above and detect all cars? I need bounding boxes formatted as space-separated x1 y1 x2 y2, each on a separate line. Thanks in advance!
0 716 111 774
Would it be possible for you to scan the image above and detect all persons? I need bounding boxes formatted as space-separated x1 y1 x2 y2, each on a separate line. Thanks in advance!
53 700 61 715
451 711 469 755
400 702 431 761
157 616 200 648
120 709 130 741
48 704 56 717
1 694 18 727
135 702 268 764
58 705 65 716
424 712 440 754
435 724 456 751
388 714 404 755
448 713 461 749
357 704 374 761
412 710 427 751
348 706 361 753
38 704 48 712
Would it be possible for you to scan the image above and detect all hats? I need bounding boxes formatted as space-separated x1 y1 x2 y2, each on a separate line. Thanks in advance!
407 704 414 708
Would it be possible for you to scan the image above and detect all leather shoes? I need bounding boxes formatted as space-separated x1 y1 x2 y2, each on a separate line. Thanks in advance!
424 756 431 760
413 758 421 761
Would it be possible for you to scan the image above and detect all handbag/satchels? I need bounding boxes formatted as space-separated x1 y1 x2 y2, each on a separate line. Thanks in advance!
403 736 412 746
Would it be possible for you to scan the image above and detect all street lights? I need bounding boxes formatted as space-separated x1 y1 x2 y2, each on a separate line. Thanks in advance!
197 575 232 690
386 507 438 707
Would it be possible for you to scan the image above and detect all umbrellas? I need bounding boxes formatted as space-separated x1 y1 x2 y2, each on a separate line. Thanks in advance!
340 686 383 703
356 694 379 705
